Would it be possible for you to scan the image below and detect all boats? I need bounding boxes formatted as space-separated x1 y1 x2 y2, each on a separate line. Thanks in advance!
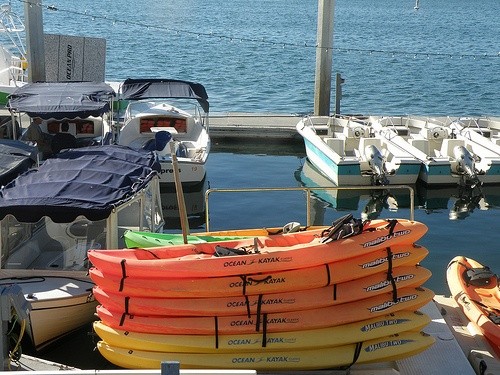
118 79 212 183
366 113 499 187
87 219 439 369
0 144 166 350
418 180 499 223
439 113 500 162
445 253 500 351
292 160 418 222
295 112 422 187
122 219 334 248
158 183 212 230
7 73 115 147
